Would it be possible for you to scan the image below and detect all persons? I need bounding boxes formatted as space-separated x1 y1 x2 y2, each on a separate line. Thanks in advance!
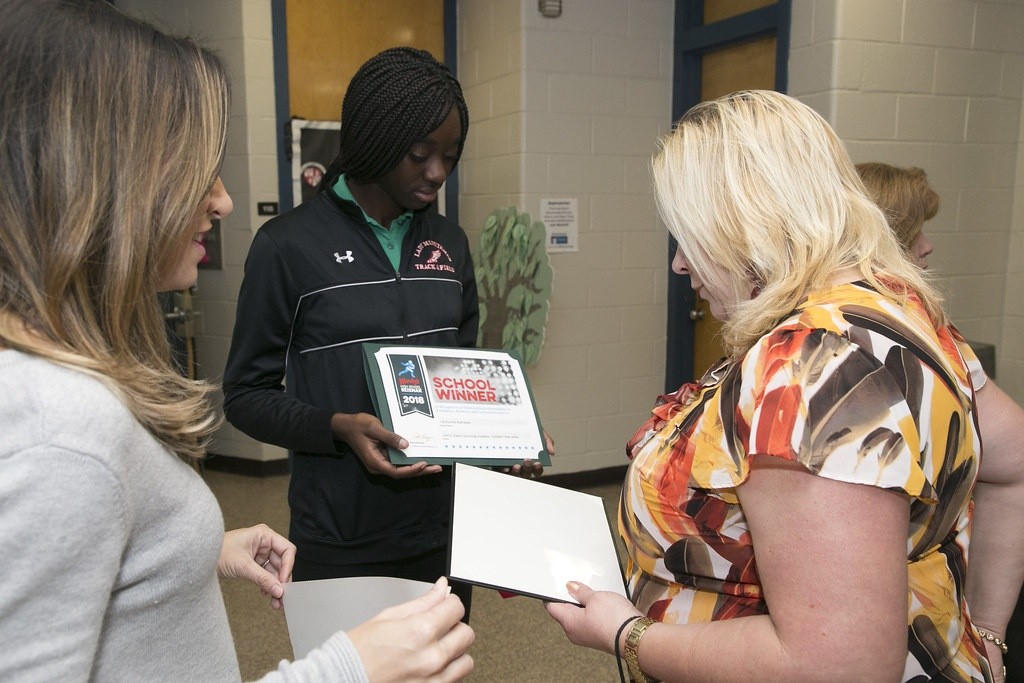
1 1 475 683
851 163 938 273
223 43 553 627
540 89 1024 683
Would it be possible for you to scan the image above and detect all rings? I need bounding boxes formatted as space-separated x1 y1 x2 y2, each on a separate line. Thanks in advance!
262 560 269 567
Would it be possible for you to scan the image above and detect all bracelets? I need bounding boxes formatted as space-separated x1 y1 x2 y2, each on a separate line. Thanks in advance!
624 617 657 683
977 629 1007 653
